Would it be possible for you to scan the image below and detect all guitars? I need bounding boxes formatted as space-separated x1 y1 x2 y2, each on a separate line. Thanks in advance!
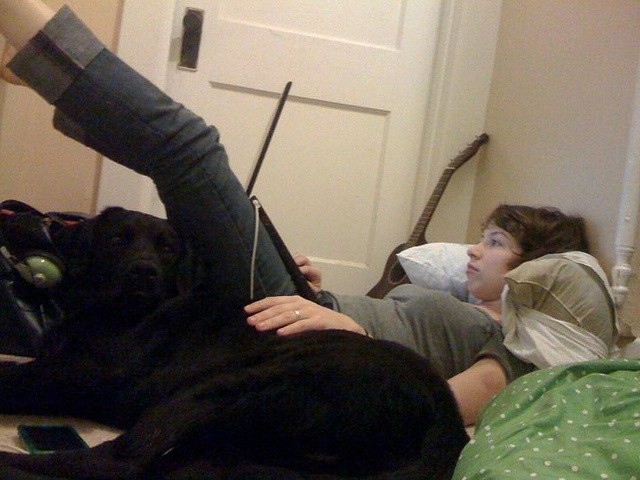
365 132 488 298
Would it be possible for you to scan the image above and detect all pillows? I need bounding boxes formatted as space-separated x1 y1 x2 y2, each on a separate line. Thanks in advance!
395 240 477 303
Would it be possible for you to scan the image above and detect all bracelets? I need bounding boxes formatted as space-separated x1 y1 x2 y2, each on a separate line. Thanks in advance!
364 327 369 336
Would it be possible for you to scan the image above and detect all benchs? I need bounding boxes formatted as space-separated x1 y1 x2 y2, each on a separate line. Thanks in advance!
501 250 617 369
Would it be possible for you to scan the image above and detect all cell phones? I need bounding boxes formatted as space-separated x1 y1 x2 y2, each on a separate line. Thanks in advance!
17 421 90 455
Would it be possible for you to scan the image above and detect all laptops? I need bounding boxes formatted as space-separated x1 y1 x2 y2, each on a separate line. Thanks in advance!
243 81 321 306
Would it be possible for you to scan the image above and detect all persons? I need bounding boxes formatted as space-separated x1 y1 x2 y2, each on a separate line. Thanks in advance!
0 1 588 428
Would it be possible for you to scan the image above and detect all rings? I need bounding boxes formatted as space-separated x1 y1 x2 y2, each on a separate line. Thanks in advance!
294 309 301 321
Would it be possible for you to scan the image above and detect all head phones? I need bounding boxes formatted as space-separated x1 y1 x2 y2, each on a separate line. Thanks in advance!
1 197 65 294
